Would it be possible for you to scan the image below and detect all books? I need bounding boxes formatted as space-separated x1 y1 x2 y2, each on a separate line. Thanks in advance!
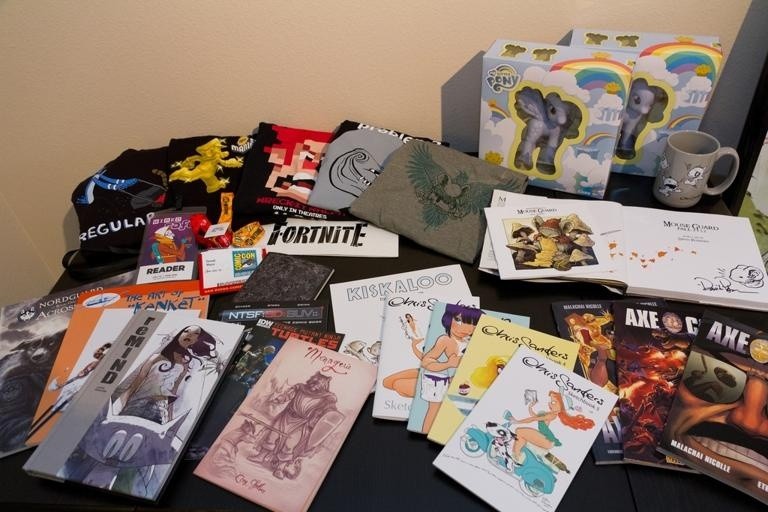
0 209 624 511
553 299 767 511
479 183 767 313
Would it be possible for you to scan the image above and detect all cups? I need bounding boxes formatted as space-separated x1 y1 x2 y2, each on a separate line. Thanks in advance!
652 131 740 208
